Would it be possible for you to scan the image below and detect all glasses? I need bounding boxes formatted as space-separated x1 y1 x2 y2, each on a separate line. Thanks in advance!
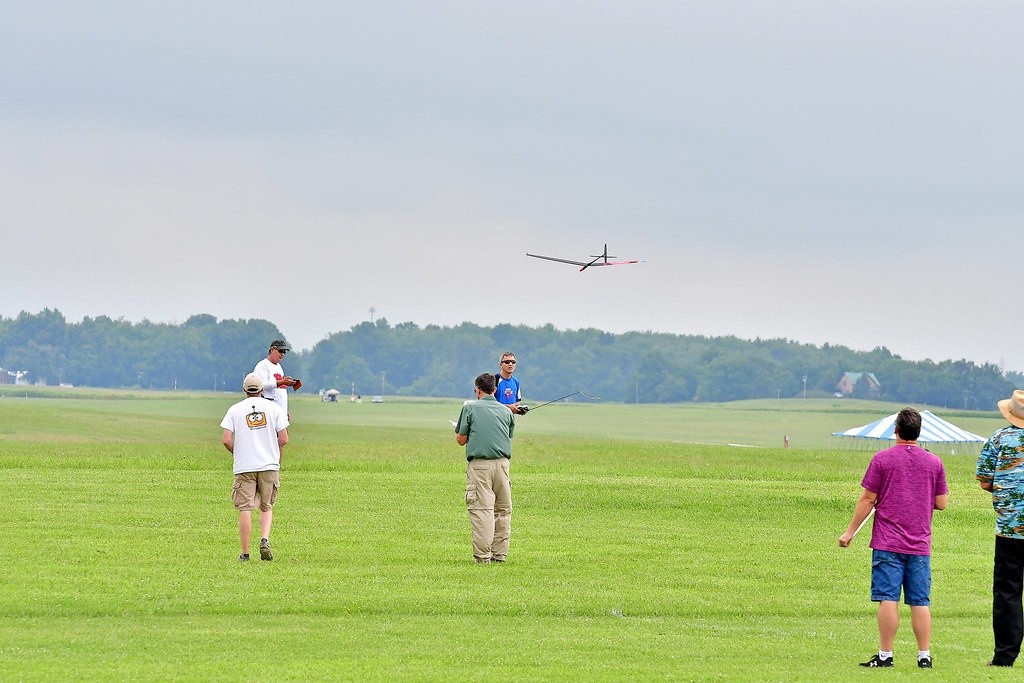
274 348 287 353
501 360 516 364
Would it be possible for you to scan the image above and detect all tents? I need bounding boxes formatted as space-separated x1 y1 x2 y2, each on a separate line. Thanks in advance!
831 410 989 452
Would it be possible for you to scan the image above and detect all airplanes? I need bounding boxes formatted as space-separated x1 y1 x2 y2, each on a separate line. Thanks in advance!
526 243 646 272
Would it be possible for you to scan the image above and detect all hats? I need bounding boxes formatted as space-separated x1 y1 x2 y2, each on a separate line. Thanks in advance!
243 372 263 393
998 390 1024 428
270 339 290 351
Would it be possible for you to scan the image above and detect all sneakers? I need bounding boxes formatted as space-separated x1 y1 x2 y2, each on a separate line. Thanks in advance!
918 658 932 668
240 554 250 560
858 653 894 668
258 538 273 561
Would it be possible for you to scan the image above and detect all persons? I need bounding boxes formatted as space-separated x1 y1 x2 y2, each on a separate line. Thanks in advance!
839 407 949 668
219 373 290 560
495 351 527 416
976 390 1024 666
253 338 297 466
455 371 516 565
